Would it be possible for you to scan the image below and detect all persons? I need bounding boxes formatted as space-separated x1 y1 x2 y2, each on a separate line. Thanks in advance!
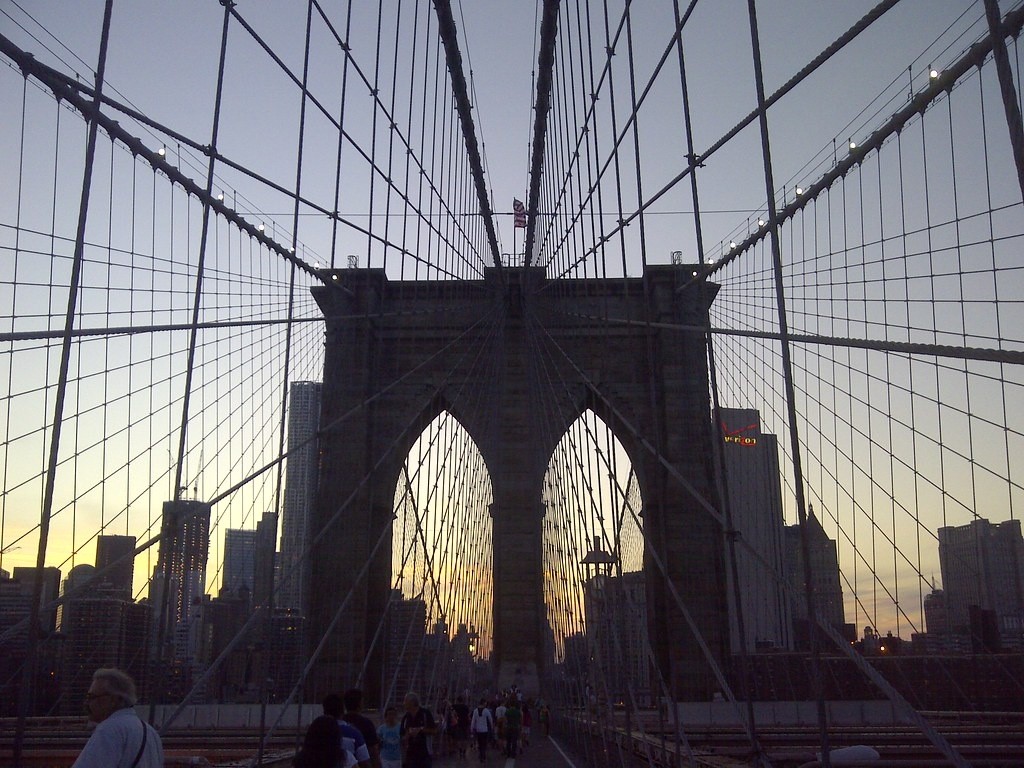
320 695 371 768
470 698 494 763
399 693 436 768
486 678 553 758
70 667 164 768
343 688 383 768
374 708 403 767
449 696 472 756
293 714 359 768
